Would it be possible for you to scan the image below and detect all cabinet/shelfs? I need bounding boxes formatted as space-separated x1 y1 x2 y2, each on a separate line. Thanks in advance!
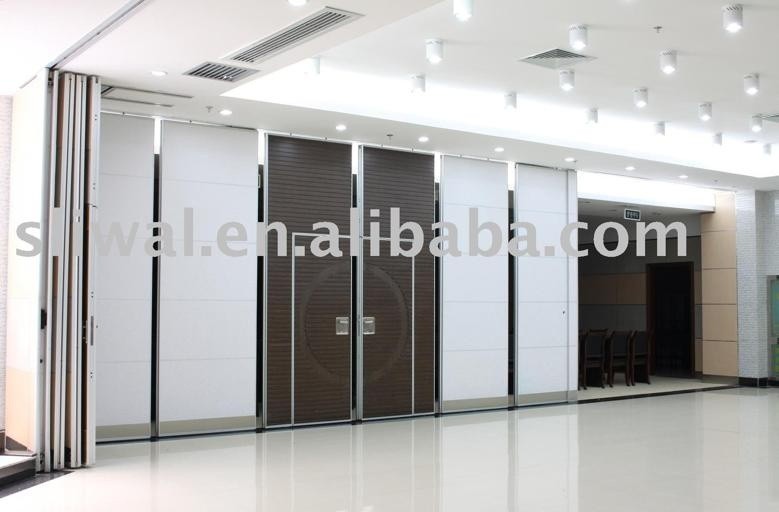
96 111 582 440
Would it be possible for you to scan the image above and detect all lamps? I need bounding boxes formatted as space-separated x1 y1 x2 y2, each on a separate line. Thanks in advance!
205 1 779 180
